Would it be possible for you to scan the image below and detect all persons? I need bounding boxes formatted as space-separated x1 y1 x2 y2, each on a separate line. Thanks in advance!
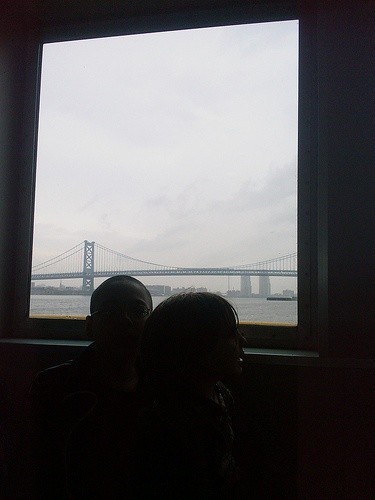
137 295 247 500
30 278 151 500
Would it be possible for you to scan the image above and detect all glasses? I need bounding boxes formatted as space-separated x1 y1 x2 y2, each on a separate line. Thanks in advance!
90 304 150 320
